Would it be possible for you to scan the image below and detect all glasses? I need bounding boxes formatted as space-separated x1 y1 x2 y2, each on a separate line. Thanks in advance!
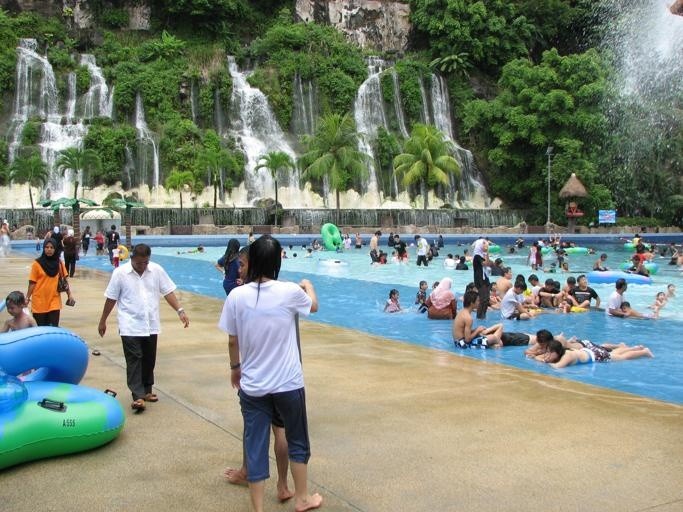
135 262 149 265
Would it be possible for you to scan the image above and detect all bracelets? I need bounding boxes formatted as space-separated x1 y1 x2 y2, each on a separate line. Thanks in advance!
25 296 30 301
175 307 185 316
229 361 241 371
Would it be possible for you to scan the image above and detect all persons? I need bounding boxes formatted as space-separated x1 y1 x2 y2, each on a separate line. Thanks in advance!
415 258 675 370
619 227 682 279
217 234 323 512
384 289 401 313
224 245 295 500
471 239 503 320
0 291 38 333
24 238 74 328
98 243 189 408
214 238 244 294
35 224 121 278
510 237 576 273
593 254 609 271
248 231 362 258
370 231 496 270
178 246 205 255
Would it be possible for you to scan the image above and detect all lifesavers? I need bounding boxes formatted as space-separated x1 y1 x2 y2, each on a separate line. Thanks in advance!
0 370 29 414
464 261 472 267
322 223 343 251
488 245 501 253
586 268 652 285
623 243 652 250
571 305 588 312
540 247 588 255
116 245 128 261
0 326 89 385
522 288 532 296
0 382 124 470
620 261 660 274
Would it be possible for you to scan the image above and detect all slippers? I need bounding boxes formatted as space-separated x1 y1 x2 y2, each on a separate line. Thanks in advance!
131 399 146 410
145 393 158 402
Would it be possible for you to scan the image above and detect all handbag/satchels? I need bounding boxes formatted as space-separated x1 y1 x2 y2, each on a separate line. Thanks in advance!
57 260 69 292
